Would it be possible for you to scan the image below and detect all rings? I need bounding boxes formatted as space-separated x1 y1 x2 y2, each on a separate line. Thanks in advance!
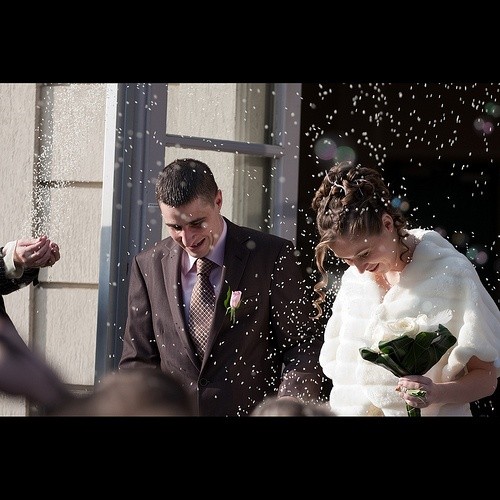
425 396 428 401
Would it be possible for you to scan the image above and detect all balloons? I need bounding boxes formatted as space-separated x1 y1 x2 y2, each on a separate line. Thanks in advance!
466 247 487 263
331 146 356 164
474 118 493 133
316 138 337 160
392 198 409 211
485 103 500 116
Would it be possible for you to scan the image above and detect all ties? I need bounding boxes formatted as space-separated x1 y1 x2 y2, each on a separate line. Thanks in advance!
186 256 219 365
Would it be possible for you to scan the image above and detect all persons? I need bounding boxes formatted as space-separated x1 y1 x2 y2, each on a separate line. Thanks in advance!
309 161 500 417
250 399 335 416
0 236 191 416
117 159 323 416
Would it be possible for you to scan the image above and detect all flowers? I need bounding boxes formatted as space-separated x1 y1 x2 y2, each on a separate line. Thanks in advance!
359 309 460 417
222 279 242 325
